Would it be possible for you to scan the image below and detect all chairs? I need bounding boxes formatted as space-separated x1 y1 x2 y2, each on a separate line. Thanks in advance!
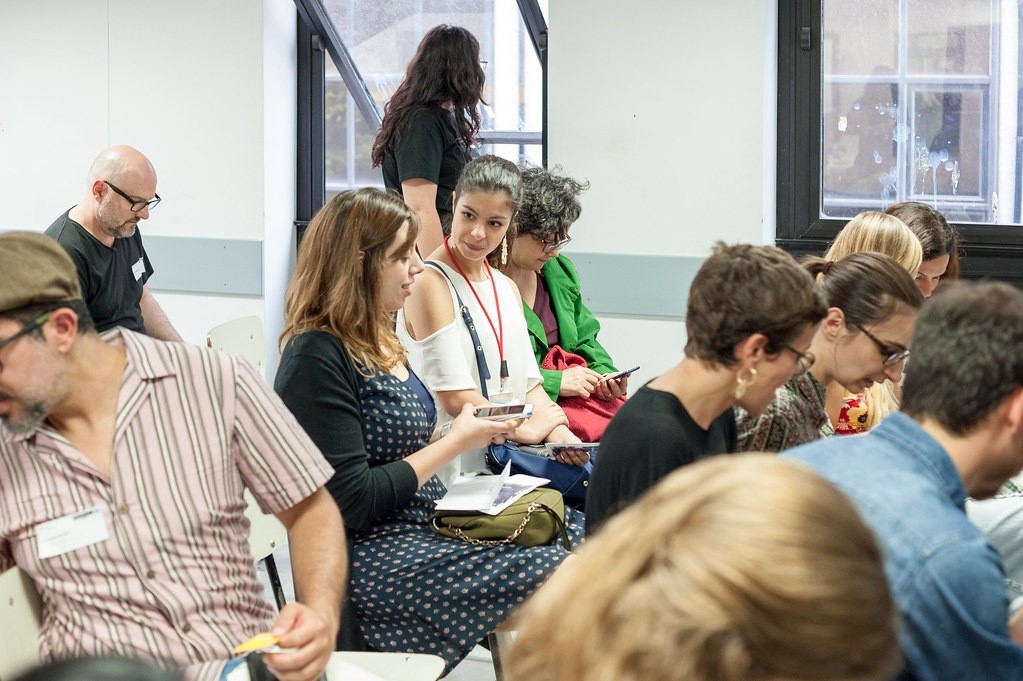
206 317 262 360
0 562 43 680
241 487 446 681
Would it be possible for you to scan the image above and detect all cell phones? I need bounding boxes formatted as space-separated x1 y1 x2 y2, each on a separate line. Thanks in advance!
474 405 534 419
605 366 640 383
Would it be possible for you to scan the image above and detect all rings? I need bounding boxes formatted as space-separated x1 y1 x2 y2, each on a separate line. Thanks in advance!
607 394 611 397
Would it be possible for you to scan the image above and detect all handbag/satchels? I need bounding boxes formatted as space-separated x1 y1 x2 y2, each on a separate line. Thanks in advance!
487 440 589 509
430 488 565 548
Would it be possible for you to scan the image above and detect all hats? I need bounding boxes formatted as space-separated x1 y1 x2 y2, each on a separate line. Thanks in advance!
0 230 83 312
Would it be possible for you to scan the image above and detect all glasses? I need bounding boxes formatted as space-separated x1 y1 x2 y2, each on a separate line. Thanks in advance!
784 343 816 377
104 180 161 212
478 60 489 70
531 231 572 254
857 324 910 367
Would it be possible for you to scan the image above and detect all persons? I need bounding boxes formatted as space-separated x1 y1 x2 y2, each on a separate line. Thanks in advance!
494 165 631 443
41 145 185 341
394 154 593 511
271 183 586 680
370 24 490 261
499 202 1023 681
0 230 347 681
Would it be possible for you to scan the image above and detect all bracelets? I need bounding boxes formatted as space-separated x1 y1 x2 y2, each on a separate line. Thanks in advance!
440 421 452 439
